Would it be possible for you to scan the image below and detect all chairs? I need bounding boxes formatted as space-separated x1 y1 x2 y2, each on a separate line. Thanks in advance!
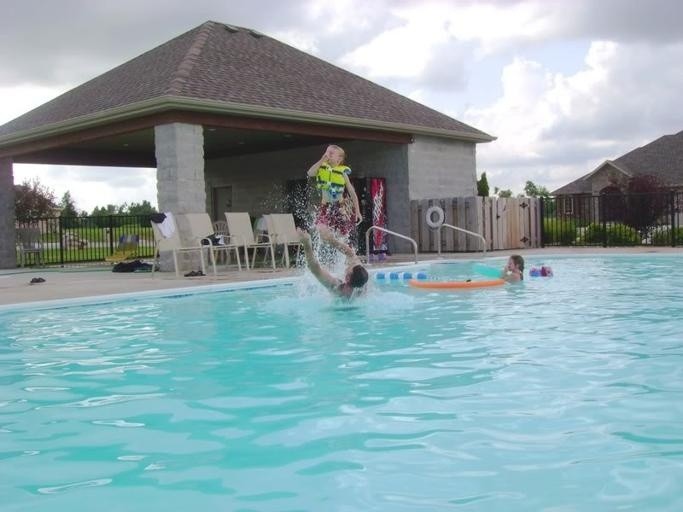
149 212 304 279
105 234 139 266
16 227 45 269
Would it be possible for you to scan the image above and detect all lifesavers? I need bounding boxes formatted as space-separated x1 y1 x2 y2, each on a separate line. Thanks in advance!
426 206 446 228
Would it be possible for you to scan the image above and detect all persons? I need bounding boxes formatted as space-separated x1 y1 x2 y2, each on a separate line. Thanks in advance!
502 254 524 285
293 222 368 301
307 144 361 271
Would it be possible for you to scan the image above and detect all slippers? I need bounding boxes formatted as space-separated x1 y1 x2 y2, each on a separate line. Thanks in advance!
183 271 206 277
30 277 45 283
